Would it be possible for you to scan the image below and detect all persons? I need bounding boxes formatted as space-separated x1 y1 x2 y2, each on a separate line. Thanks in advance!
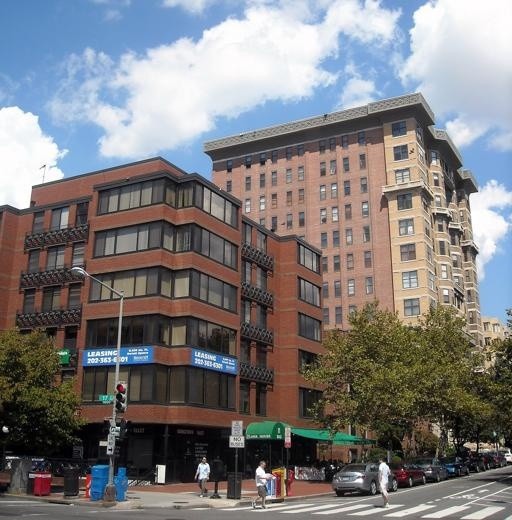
193 456 211 498
377 455 392 507
251 461 276 510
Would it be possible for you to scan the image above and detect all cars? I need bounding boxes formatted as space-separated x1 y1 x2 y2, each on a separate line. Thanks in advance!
330 448 512 499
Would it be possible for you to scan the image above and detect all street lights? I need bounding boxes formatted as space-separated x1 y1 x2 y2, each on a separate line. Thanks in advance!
70 266 126 506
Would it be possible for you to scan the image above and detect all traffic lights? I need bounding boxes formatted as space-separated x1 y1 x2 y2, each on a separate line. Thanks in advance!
115 380 127 414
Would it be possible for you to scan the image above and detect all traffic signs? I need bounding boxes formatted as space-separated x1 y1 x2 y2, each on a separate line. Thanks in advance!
107 434 115 455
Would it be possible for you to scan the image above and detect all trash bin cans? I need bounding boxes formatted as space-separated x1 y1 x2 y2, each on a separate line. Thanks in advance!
227 472 241 498
63 466 80 496
